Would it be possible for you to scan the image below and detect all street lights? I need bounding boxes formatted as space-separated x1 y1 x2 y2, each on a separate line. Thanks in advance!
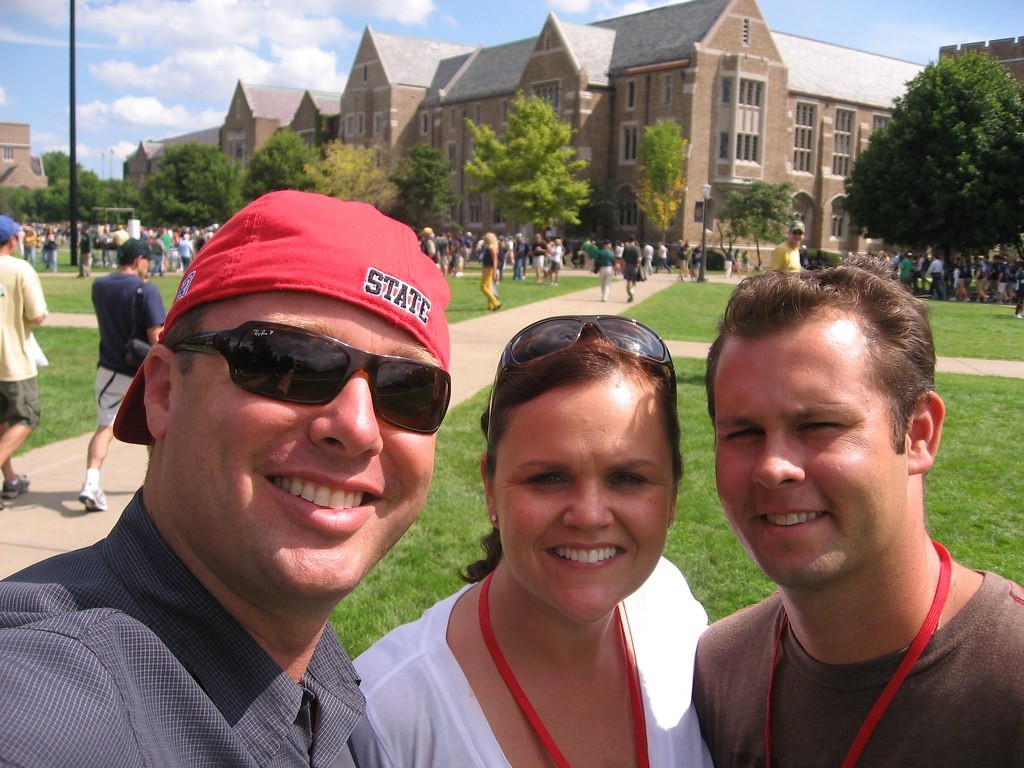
696 184 713 283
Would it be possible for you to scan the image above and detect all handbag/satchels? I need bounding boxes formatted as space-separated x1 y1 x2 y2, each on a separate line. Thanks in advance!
122 336 150 369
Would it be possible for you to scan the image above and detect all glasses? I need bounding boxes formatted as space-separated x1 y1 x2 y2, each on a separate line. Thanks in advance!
168 319 452 434
484 314 677 456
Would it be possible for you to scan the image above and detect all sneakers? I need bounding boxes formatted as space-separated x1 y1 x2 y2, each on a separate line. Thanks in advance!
80 486 106 512
2 476 30 498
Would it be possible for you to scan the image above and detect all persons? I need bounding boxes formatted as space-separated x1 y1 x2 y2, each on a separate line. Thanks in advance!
419 227 566 313
12 220 70 272
946 252 1024 319
1 189 451 768
0 216 48 511
352 316 715 768
76 223 129 278
78 237 166 511
566 235 750 303
770 219 805 273
845 250 946 301
140 223 220 278
691 264 1023 768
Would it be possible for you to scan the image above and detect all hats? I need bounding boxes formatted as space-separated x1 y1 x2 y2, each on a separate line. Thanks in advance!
0 215 20 242
112 188 449 445
117 236 162 267
